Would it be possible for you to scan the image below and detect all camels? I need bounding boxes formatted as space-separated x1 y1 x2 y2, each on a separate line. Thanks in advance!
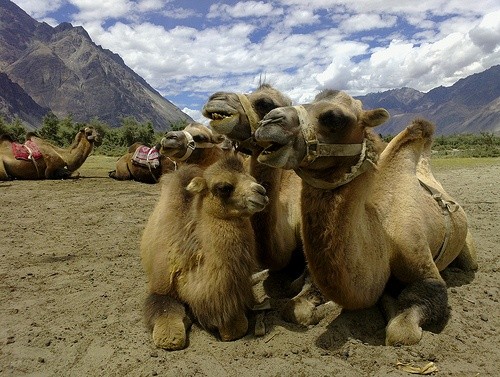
108 142 175 183
139 152 270 352
255 88 478 345
202 84 306 301
0 127 102 181
158 122 226 171
